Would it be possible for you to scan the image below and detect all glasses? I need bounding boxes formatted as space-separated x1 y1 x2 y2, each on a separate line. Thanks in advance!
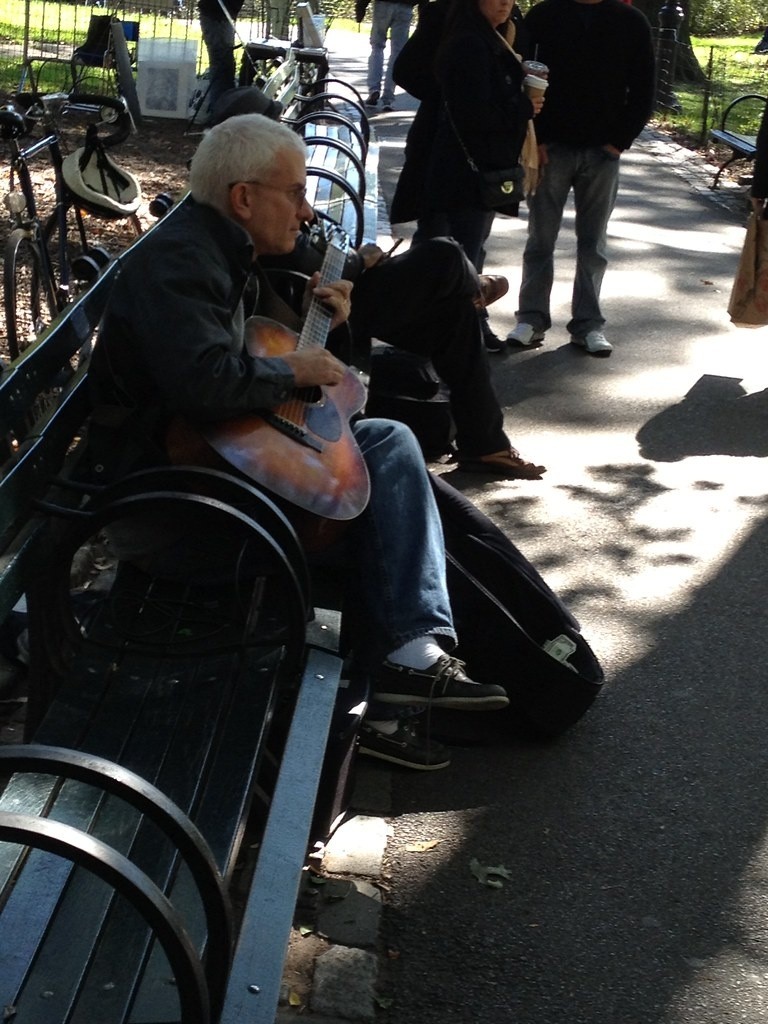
229 178 306 210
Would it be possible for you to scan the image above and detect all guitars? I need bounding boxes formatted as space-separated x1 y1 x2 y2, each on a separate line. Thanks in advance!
164 219 374 556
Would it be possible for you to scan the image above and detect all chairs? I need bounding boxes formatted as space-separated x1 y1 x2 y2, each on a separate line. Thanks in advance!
65 16 141 98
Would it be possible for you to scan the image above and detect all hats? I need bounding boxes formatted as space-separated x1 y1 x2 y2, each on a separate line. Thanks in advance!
210 85 284 129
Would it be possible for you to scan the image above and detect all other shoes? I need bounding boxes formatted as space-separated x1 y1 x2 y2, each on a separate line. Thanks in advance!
456 445 544 479
366 92 380 107
471 274 508 312
382 99 392 111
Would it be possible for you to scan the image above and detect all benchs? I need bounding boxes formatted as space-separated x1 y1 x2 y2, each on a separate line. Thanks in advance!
706 90 767 189
1 56 391 1024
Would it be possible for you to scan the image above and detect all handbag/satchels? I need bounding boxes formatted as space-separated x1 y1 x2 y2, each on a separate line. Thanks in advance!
729 198 768 325
479 166 527 208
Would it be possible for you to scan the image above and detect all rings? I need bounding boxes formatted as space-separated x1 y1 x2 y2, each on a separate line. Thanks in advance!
343 299 347 304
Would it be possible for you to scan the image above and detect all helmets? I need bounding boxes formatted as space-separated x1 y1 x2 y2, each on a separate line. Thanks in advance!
58 146 142 220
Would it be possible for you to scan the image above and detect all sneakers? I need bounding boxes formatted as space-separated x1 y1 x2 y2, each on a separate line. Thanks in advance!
570 325 614 356
374 651 509 710
347 707 454 769
506 322 545 347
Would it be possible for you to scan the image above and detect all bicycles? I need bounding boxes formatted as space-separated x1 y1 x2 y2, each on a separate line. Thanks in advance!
0 84 144 363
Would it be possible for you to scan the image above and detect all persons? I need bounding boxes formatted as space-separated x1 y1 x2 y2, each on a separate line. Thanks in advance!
80 112 512 770
208 88 545 480
750 98 768 220
198 0 245 107
388 0 655 353
354 0 419 112
